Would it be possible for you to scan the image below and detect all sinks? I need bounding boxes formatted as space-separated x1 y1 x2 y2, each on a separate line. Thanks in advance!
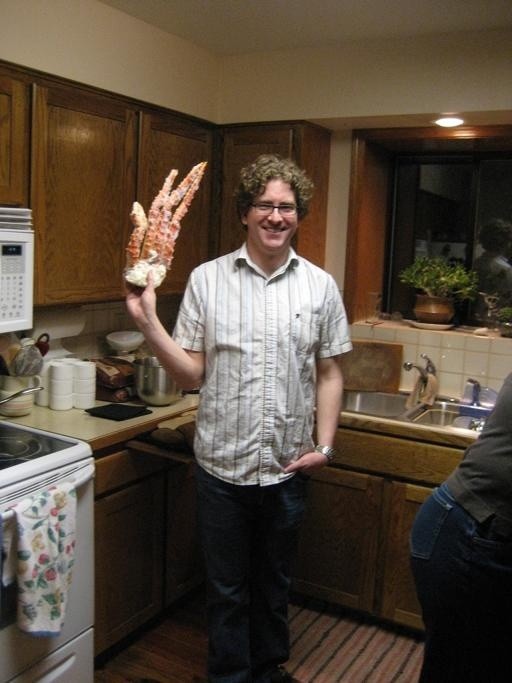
404 401 496 438
339 390 422 421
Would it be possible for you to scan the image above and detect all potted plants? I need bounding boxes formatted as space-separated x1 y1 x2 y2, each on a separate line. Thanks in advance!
398 253 486 323
494 308 512 338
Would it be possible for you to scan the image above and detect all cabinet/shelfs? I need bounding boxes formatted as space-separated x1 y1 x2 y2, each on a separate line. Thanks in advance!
0 55 218 312
287 407 482 643
215 121 334 277
84 404 226 667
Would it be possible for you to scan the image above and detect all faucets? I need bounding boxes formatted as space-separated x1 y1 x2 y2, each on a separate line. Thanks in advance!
466 377 481 406
404 363 435 407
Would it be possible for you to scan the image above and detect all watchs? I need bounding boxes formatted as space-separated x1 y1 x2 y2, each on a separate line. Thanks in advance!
314 445 337 461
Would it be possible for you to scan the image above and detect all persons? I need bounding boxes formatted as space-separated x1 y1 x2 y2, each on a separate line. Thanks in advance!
469 220 511 297
410 364 512 682
125 158 352 683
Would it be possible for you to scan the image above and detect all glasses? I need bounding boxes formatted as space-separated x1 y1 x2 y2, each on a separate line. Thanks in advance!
253 203 299 214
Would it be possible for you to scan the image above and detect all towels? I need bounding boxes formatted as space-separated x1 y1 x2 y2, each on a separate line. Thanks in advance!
2 481 78 637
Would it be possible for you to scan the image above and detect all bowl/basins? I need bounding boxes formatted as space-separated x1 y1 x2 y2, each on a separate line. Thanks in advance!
132 356 183 407
106 330 146 353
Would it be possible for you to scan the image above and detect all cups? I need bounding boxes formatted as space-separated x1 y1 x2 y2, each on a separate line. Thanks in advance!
72 394 96 411
50 361 75 381
74 378 96 393
35 390 50 408
34 361 49 377
34 377 51 392
74 362 97 380
49 393 74 411
50 379 74 395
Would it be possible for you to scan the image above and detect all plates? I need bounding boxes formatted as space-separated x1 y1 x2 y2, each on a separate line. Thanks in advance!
402 318 455 331
